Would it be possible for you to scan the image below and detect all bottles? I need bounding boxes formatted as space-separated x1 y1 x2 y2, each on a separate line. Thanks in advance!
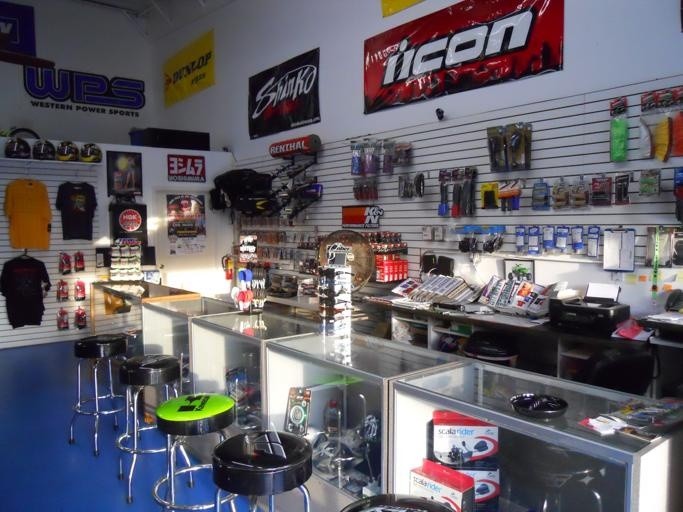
325 400 342 435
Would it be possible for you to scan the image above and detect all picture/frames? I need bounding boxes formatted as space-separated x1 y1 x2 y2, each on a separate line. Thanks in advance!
504 259 535 285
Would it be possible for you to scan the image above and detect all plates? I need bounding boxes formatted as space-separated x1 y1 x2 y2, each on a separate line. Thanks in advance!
510 394 568 418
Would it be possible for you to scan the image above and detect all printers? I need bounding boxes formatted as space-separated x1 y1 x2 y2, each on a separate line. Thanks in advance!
548 282 630 331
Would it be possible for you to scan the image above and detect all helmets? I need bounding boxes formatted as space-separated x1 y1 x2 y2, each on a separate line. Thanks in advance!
4 137 102 162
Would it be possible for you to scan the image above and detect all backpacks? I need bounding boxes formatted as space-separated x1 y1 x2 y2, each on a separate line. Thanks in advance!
211 169 271 216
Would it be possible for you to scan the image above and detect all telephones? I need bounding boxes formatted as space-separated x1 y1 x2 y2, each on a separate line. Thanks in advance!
664 290 683 311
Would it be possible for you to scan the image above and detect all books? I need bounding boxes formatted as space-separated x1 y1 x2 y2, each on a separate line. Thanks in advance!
391 275 578 317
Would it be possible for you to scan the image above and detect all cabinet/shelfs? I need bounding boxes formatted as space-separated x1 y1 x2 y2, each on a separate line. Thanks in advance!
91 279 683 511
237 224 321 313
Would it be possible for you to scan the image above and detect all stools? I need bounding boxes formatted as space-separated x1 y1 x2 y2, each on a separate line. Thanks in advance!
69 333 129 456
